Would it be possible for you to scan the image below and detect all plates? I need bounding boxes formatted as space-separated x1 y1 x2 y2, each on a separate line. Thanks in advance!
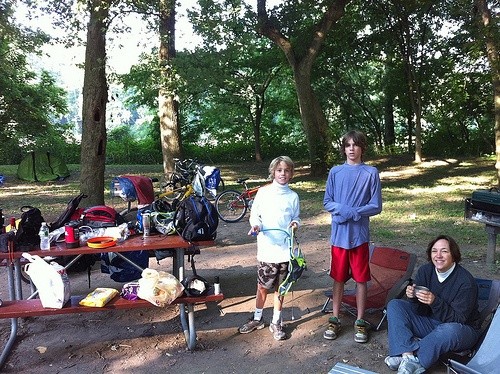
86 237 117 248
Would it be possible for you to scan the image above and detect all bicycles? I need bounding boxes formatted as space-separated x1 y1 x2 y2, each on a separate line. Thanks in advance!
112 158 227 229
215 178 263 223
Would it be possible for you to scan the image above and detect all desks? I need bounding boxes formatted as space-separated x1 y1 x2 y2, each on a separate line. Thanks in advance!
0 231 216 372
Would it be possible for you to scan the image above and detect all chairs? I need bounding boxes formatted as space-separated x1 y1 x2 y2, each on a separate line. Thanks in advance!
414 278 500 374
320 245 416 331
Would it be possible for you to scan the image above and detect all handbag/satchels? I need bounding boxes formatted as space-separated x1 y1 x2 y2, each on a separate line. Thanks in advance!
16 205 44 251
23 253 71 309
137 268 183 308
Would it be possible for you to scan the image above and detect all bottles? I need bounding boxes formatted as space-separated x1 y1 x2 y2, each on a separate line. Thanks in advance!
8 218 18 237
141 212 151 236
39 222 51 251
213 276 220 295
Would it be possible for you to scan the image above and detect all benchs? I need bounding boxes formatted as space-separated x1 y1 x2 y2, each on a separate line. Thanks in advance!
0 246 224 320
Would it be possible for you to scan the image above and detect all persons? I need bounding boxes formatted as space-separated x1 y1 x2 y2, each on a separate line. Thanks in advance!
237 156 301 340
384 235 481 374
323 130 382 342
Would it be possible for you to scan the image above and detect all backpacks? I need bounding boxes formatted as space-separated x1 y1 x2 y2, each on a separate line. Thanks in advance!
150 195 219 242
49 192 89 232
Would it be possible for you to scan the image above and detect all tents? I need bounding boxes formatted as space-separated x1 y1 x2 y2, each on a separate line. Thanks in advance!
17 151 70 182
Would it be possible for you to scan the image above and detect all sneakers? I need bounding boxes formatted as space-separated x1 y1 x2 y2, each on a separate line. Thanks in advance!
398 355 426 374
353 323 368 342
269 321 286 341
385 355 402 371
239 316 265 334
324 320 342 340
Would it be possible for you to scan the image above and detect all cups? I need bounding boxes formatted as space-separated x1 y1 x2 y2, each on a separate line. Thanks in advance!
414 286 429 296
65 222 79 248
